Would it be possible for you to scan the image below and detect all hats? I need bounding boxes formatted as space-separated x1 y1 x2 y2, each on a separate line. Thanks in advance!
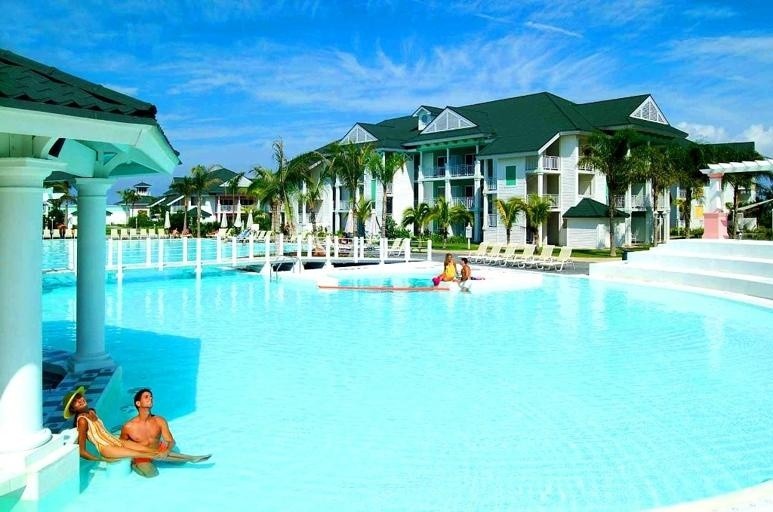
63 385 84 419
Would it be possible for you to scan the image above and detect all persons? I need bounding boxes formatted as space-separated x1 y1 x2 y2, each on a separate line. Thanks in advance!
432 253 471 293
63 385 212 478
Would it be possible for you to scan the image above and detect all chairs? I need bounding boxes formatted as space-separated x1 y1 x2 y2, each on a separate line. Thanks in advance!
214 228 272 243
42 228 77 239
459 241 575 273
387 237 411 257
110 228 168 240
